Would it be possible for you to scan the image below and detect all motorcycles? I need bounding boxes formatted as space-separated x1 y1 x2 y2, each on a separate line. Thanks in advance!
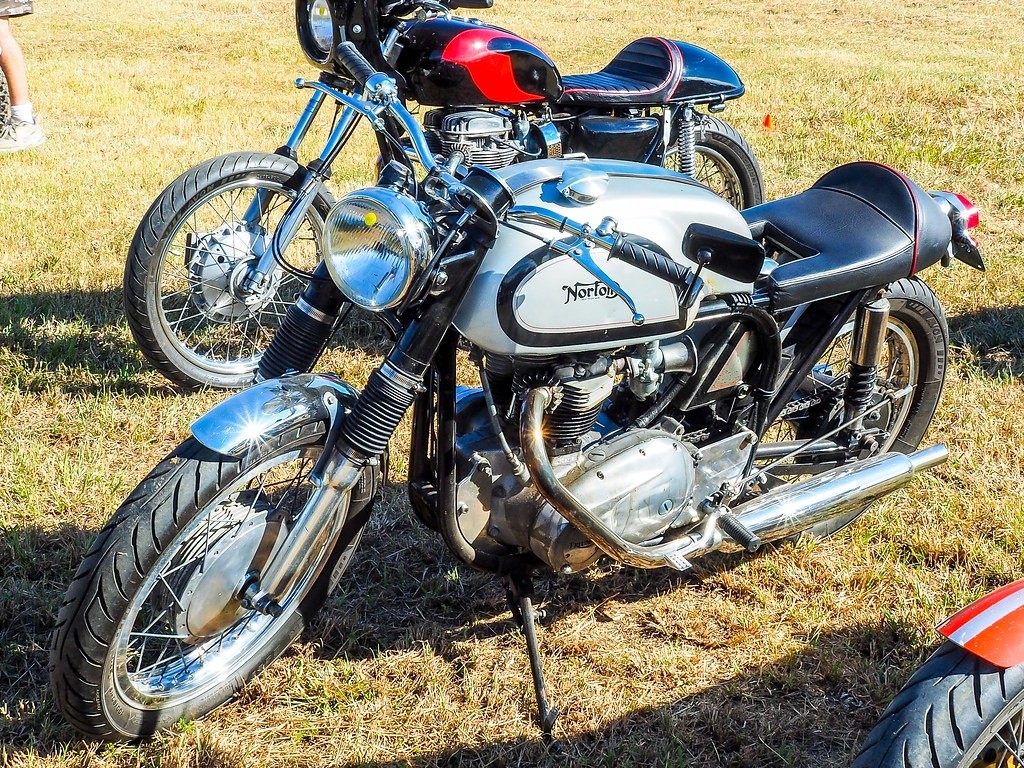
42 42 989 745
117 0 767 397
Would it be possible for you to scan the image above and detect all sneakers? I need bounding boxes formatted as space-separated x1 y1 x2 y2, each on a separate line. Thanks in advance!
0 117 45 153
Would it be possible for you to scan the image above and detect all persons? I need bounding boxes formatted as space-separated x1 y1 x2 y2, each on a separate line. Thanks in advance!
0 0 47 154
